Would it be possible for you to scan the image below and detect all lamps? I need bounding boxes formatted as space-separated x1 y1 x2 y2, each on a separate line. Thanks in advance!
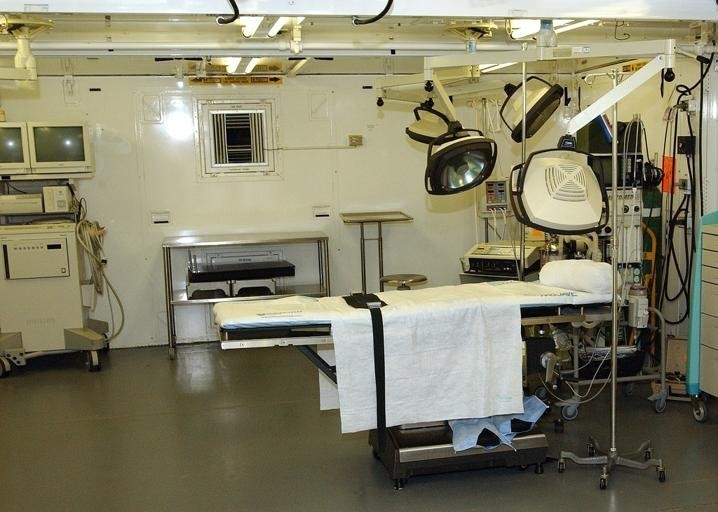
424 119 498 195
406 104 460 144
500 79 564 144
510 132 610 235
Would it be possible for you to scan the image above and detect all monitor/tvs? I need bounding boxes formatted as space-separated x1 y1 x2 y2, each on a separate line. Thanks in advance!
0 120 31 175
27 120 96 173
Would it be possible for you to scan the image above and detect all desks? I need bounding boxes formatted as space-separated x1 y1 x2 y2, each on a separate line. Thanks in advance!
162 231 329 358
341 212 414 293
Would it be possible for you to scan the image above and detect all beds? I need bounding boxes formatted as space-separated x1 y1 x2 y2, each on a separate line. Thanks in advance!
214 279 630 490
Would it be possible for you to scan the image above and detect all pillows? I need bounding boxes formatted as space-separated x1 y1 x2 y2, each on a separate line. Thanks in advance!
539 258 623 292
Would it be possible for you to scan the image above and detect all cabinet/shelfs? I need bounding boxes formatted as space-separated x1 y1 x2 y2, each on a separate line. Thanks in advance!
685 210 718 399
